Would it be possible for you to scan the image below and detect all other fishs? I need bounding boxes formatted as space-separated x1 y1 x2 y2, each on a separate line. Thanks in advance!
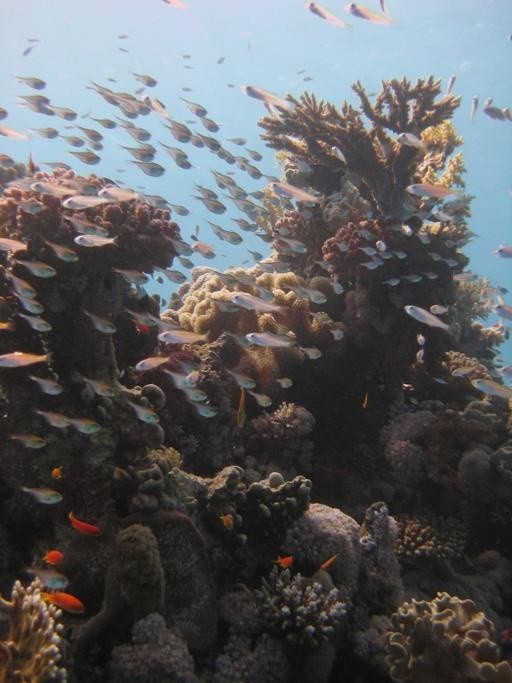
0 0 512 509
